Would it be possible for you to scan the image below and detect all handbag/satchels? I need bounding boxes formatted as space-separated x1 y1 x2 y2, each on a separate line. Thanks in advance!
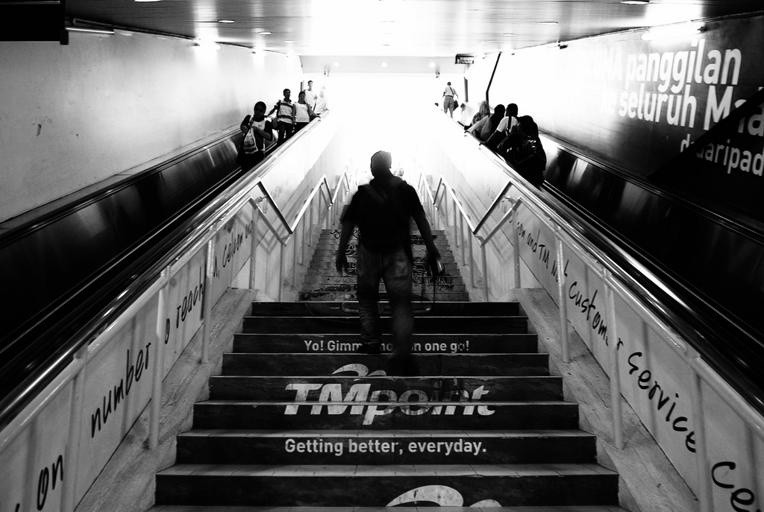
273 118 278 129
506 140 536 163
235 146 245 164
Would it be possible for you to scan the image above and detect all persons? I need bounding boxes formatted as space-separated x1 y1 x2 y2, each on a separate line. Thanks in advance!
302 80 317 121
292 91 320 136
461 100 546 189
236 102 273 174
335 150 441 375
268 88 295 148
441 81 458 119
